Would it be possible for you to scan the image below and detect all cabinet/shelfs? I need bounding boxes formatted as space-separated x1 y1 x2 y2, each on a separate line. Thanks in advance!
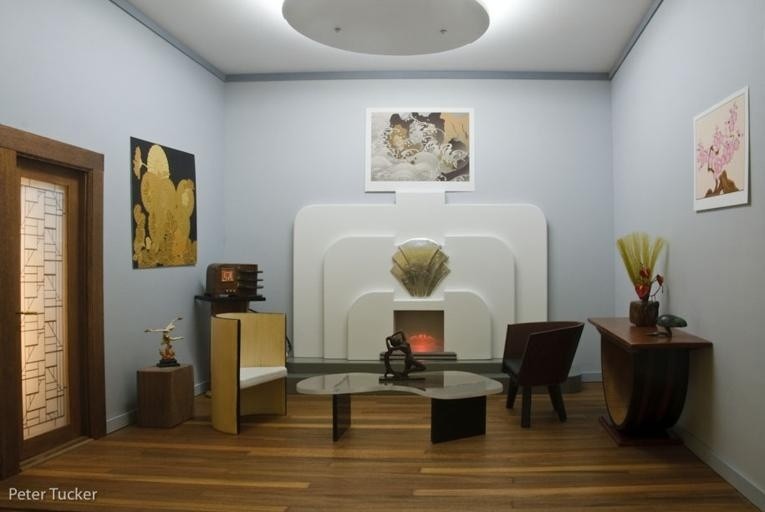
135 362 195 428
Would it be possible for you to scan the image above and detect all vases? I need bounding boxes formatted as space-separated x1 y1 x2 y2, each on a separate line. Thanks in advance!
629 300 661 328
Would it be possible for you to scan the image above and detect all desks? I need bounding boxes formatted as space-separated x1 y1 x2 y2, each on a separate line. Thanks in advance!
195 296 266 400
588 312 716 449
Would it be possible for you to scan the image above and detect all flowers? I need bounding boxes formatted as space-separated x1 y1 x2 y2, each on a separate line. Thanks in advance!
616 229 667 302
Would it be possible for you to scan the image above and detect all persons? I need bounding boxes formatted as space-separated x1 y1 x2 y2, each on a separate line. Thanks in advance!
385 327 426 379
142 316 185 348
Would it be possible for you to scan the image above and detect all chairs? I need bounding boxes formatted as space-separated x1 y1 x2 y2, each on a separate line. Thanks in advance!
500 318 585 430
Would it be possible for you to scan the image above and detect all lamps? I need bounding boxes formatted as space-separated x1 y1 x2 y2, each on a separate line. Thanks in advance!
279 0 490 57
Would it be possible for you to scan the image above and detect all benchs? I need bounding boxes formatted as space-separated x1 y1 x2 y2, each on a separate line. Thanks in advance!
211 308 288 436
